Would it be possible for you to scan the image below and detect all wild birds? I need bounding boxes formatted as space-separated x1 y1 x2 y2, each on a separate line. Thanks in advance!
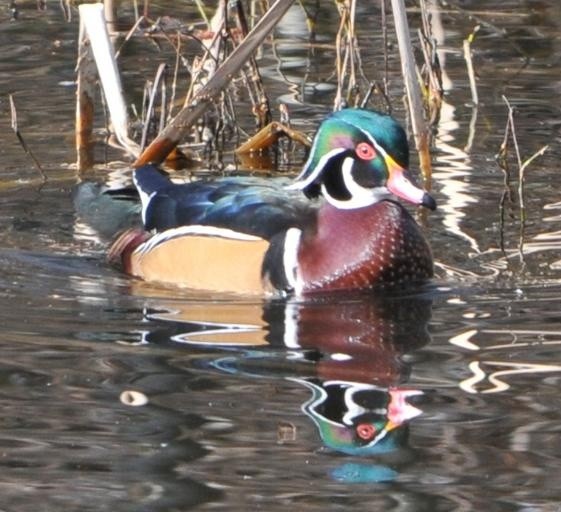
72 105 437 294
32 277 439 457
